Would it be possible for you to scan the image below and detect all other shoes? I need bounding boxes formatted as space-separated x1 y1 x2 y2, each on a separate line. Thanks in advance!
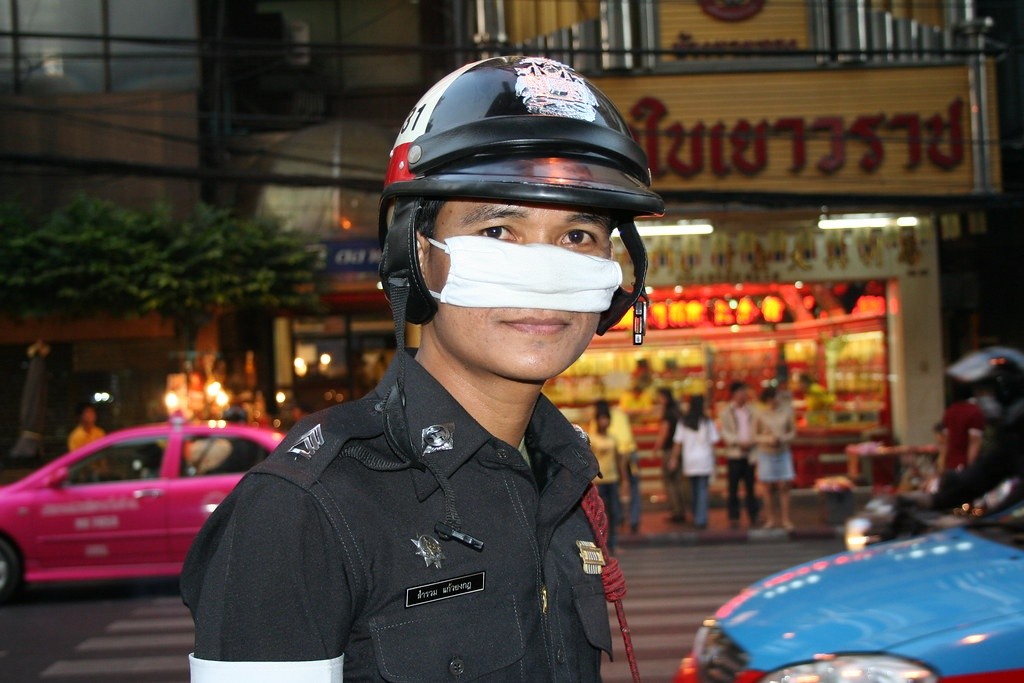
762 519 780 530
692 520 707 528
749 516 767 530
782 520 795 531
665 515 685 523
631 522 637 533
618 517 625 527
728 520 740 532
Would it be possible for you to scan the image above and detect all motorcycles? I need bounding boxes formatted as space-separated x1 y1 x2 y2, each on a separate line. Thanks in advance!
842 472 1023 546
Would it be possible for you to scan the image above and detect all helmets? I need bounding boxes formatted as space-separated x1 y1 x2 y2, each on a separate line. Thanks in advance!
377 55 665 337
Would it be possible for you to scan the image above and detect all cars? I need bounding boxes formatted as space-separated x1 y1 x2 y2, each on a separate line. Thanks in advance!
675 501 1021 683
0 421 285 606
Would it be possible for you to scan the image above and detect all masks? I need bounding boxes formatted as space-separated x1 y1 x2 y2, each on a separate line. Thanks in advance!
419 237 621 313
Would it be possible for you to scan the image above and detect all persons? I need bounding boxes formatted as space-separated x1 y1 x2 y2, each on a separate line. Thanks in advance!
586 347 1024 557
67 403 105 451
174 57 665 683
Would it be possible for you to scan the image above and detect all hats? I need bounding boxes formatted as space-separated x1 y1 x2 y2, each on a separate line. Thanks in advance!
948 346 1024 381
